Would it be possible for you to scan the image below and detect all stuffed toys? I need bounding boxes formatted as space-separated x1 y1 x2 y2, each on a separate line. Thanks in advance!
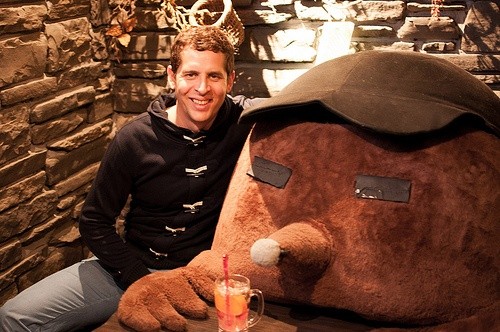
88 48 500 332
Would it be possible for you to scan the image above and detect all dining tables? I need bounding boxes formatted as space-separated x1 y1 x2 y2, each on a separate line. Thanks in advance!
77 299 374 332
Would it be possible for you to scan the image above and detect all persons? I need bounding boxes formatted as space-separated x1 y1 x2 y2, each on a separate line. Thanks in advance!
0 24 274 332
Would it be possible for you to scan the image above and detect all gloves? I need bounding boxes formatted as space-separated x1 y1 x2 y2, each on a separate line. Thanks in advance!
113 258 151 292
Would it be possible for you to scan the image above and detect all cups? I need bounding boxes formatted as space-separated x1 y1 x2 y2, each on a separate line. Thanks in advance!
213 274 265 332
